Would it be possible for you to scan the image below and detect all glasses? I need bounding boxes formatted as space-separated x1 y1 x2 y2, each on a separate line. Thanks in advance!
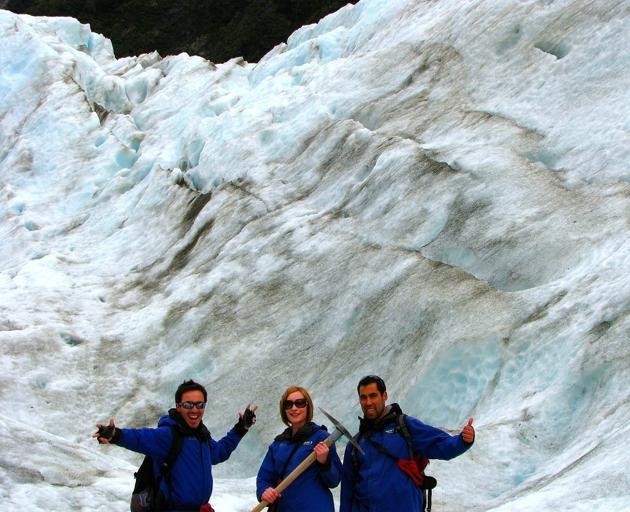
283 399 309 409
178 401 206 409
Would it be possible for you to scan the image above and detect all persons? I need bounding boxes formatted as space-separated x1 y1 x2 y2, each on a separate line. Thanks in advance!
256 386 343 512
338 375 477 511
92 379 259 512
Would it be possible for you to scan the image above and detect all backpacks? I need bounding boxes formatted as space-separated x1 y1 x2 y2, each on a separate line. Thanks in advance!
130 423 185 512
